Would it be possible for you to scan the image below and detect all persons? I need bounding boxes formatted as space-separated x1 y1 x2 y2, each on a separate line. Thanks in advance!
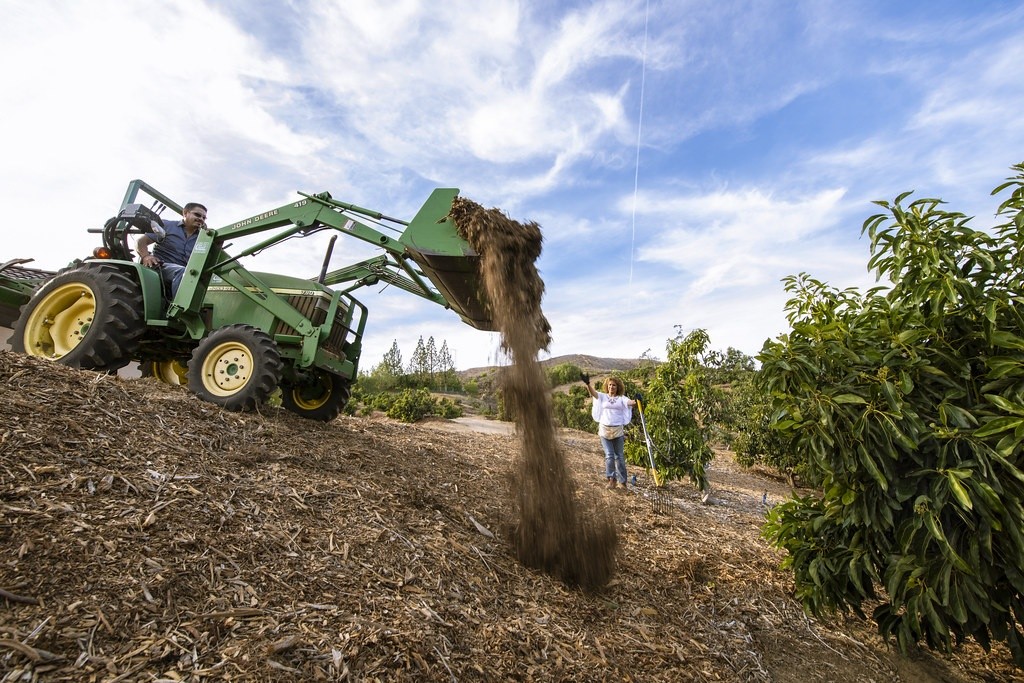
580 370 643 491
137 203 209 298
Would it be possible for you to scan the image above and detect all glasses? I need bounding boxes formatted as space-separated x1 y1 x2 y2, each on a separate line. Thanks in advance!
187 209 207 220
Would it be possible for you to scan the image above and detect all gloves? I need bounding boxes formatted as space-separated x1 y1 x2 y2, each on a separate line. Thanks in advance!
580 372 590 384
634 393 642 404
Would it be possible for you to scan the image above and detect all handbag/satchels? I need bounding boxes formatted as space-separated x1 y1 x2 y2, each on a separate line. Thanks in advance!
601 424 623 440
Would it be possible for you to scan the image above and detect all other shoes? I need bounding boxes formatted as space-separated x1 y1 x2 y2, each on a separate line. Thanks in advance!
606 477 616 489
620 483 627 489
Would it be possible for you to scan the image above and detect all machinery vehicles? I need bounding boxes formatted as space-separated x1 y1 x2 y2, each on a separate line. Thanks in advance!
0 187 506 421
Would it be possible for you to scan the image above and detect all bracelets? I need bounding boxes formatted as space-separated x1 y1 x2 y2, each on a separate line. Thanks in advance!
141 253 151 260
